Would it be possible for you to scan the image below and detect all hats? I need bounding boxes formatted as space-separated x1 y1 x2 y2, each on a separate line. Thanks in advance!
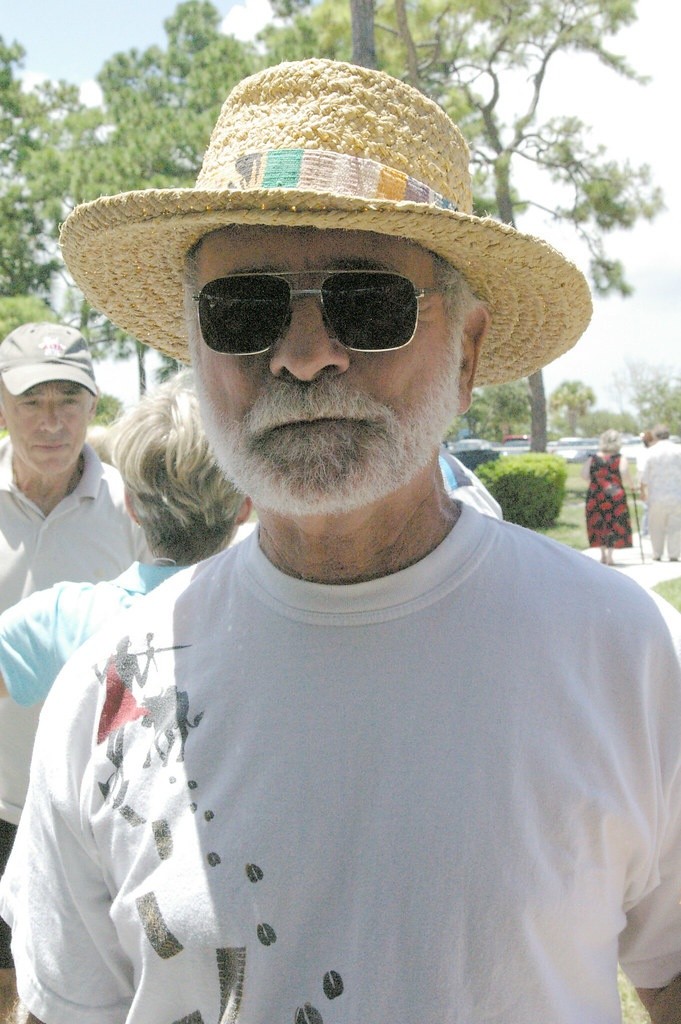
60 57 594 388
0 321 99 397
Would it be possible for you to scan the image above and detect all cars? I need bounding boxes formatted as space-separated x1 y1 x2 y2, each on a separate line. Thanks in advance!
442 433 681 472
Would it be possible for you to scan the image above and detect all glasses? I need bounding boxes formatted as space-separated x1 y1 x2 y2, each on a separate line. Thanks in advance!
182 264 457 356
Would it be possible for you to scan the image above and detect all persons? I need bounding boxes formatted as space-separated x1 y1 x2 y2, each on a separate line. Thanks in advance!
1 322 161 1024
1 56 681 1024
581 422 681 565
1 366 255 710
